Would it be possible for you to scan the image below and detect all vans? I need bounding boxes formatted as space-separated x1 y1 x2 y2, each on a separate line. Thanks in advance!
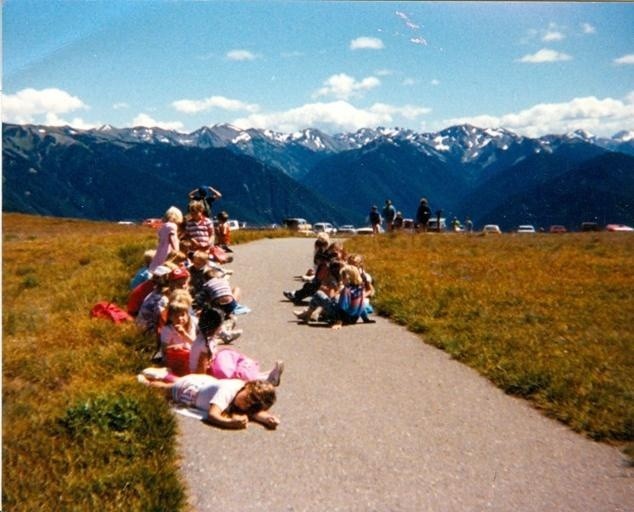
227 220 240 231
427 217 446 232
142 217 160 229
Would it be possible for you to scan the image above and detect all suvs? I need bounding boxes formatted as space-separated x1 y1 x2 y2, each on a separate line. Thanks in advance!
284 217 312 233
581 216 600 230
312 222 337 236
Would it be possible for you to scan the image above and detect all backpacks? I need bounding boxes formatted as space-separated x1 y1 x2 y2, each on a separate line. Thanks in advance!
207 349 260 383
200 196 212 218
90 300 134 327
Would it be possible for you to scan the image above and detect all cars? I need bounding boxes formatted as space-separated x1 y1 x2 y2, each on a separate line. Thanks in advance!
516 225 535 233
337 224 356 235
607 223 633 232
482 224 501 237
549 225 567 233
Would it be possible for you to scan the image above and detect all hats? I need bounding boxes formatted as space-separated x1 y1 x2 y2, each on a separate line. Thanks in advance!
168 268 191 281
199 184 209 191
218 210 229 219
153 265 173 277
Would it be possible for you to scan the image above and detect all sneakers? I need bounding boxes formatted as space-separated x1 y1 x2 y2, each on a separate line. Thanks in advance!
283 290 301 305
220 331 241 345
267 360 284 387
136 364 169 384
291 309 311 323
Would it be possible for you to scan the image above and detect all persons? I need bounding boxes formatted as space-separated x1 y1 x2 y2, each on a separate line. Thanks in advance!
126 184 284 430
284 197 432 330
452 214 475 233
